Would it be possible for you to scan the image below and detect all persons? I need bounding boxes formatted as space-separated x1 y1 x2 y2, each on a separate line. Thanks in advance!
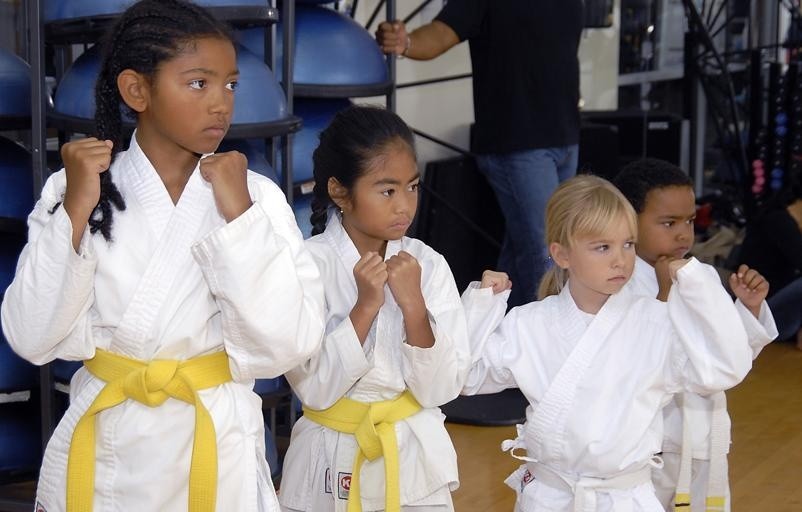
376 1 616 308
613 154 782 512
736 177 801 354
459 171 753 512
277 104 463 510
2 0 327 511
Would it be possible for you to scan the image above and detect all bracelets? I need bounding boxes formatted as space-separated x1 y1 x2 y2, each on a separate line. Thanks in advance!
395 36 413 59
